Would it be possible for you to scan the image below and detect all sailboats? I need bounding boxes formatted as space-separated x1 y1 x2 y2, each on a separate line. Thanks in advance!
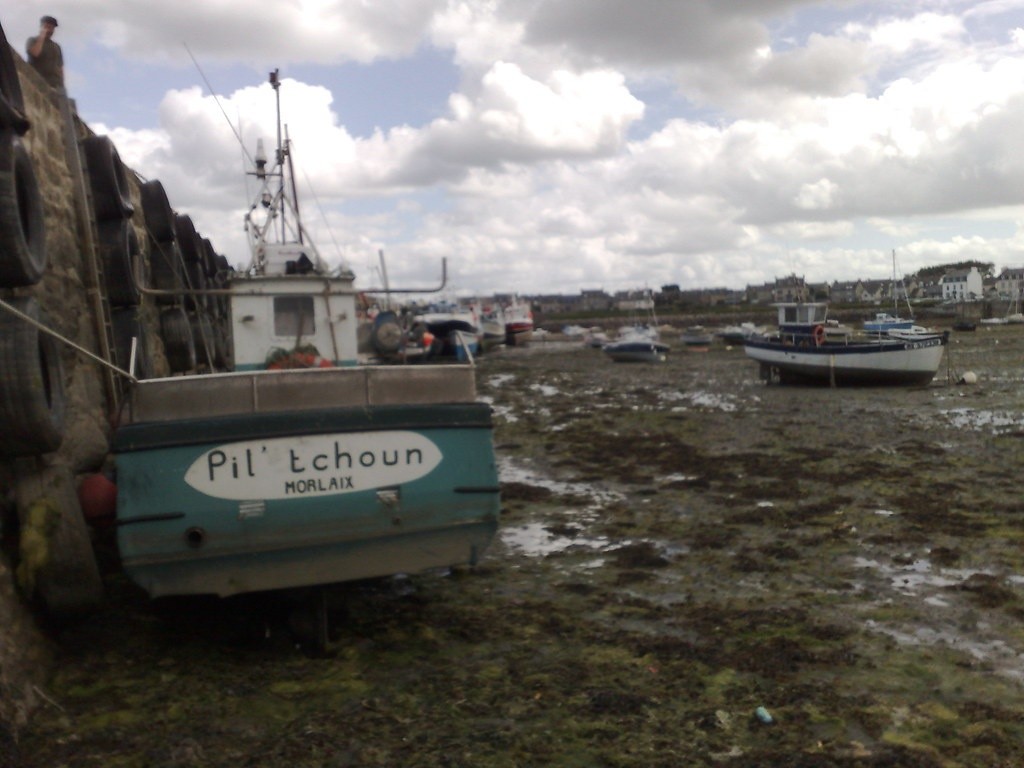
1002 286 1024 324
862 248 918 336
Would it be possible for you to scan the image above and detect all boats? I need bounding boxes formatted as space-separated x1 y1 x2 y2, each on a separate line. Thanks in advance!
978 315 1012 325
372 246 535 363
681 323 714 343
739 246 949 383
716 326 776 344
602 329 672 362
101 65 506 612
887 324 948 342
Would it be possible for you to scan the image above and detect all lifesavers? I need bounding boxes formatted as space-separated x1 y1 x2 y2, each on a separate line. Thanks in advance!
0 22 32 137
140 179 233 373
85 134 135 218
0 130 48 289
100 214 146 308
0 296 67 454
813 325 825 344
264 353 335 370
